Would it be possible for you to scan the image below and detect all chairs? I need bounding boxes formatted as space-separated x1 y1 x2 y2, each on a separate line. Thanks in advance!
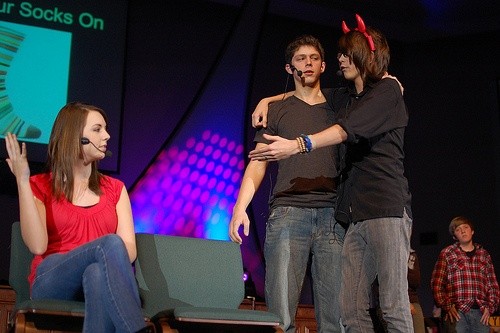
4 221 285 333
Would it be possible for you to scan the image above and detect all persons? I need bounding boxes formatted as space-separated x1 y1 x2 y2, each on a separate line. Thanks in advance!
431 216 500 333
248 14 416 333
6 102 154 333
230 34 404 333
371 246 441 333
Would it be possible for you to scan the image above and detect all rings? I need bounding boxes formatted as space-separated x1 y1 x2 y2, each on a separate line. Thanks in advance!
264 156 267 161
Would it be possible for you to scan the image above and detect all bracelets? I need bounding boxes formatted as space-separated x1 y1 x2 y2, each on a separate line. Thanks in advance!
294 134 312 154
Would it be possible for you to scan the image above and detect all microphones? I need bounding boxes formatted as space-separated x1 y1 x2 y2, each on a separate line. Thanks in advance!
80 138 112 159
290 64 302 76
335 70 343 76
